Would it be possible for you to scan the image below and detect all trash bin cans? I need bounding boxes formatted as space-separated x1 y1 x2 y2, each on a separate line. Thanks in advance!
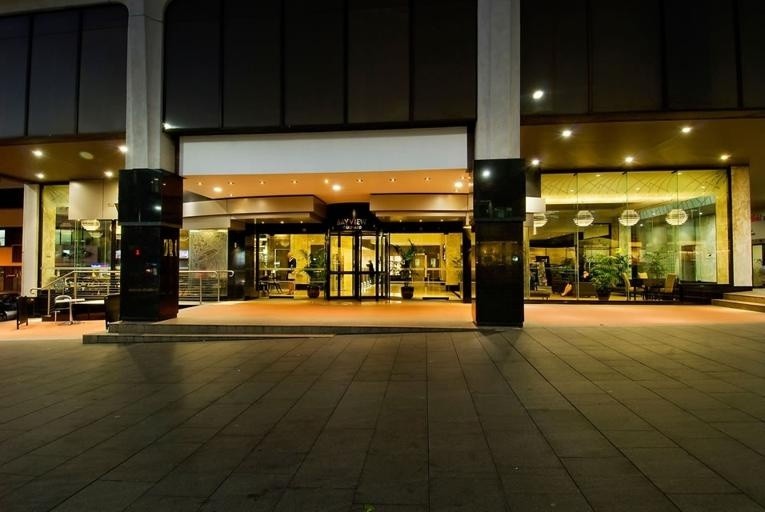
104 293 120 330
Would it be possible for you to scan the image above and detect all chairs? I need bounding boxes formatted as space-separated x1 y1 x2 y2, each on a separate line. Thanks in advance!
621 272 637 301
55 295 72 324
660 273 677 301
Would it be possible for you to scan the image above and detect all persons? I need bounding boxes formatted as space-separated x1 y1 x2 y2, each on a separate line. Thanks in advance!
560 269 590 296
365 259 376 285
286 251 296 296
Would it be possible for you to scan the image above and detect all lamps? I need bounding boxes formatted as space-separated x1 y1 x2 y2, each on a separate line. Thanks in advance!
534 203 688 228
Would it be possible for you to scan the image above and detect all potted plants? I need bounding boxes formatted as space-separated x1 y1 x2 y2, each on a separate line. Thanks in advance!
304 248 326 298
398 244 418 300
639 252 666 280
587 255 627 301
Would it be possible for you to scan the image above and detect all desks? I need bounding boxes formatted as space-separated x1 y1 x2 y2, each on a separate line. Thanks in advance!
643 279 664 301
55 299 86 324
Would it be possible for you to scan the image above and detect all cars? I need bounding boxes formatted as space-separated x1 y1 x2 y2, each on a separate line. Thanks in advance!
0 292 21 322
